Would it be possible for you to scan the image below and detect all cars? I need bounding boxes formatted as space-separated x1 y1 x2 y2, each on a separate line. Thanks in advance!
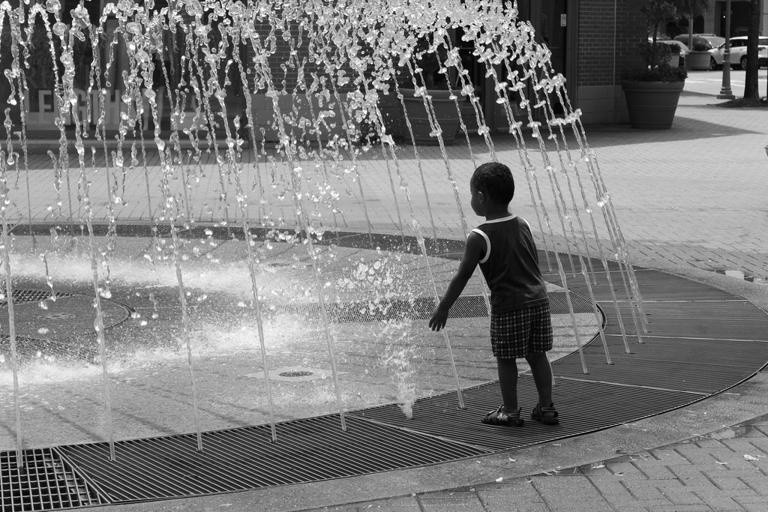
673 32 725 70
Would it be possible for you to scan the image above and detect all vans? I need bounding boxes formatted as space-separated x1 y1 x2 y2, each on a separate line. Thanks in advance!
707 34 768 70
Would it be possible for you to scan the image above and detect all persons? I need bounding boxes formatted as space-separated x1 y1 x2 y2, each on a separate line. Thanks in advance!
428 161 562 428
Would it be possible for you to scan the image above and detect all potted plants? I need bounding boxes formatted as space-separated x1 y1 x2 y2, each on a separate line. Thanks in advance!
621 0 689 130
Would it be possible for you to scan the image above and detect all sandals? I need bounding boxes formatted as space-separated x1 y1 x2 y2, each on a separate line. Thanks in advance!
530 401 560 426
480 405 524 426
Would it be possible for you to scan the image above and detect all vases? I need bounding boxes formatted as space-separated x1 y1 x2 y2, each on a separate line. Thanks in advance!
395 88 467 146
